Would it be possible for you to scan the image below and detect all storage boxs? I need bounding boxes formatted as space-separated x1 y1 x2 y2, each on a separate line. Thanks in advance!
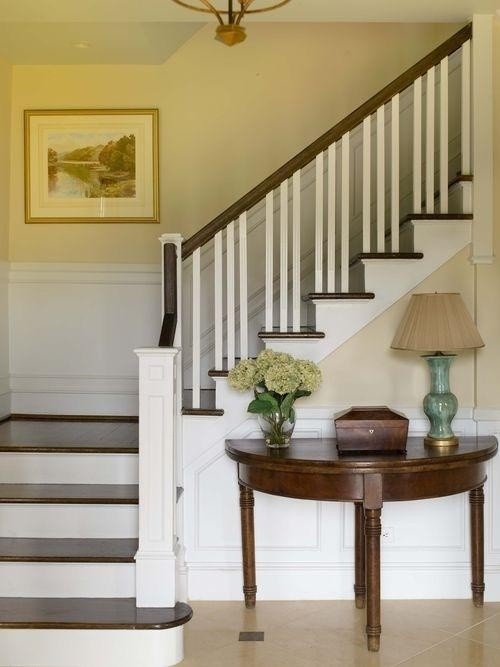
333 407 410 457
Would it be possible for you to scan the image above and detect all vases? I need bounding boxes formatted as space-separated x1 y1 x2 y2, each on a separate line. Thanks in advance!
254 403 296 448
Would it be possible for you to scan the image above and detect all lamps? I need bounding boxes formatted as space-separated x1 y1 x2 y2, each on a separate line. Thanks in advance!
173 0 290 46
390 292 485 447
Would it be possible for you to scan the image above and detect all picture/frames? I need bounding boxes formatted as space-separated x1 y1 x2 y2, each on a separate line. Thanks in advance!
23 109 160 225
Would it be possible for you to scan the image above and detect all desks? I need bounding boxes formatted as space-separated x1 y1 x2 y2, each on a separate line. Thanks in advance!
224 437 499 653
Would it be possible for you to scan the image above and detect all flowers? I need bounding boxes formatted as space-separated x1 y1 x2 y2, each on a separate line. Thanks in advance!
228 349 323 415
